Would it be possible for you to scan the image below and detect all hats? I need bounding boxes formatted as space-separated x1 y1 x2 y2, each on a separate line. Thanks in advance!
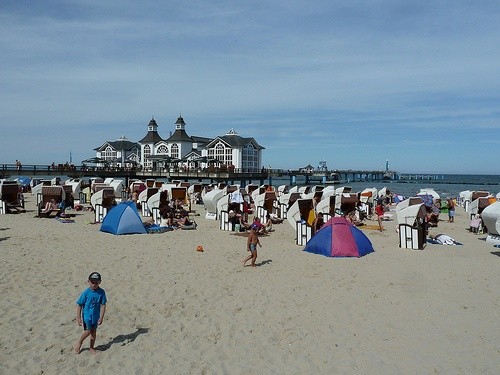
89 272 101 281
251 224 258 229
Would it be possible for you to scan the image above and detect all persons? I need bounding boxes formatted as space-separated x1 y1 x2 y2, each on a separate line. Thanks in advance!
75 272 107 355
159 201 192 227
388 192 393 197
15 160 22 170
241 214 273 235
434 199 440 209
143 219 156 228
51 162 55 171
375 200 385 232
314 212 323 234
445 197 455 222
44 201 58 211
66 162 68 164
379 195 391 212
346 211 367 228
312 196 319 211
131 190 138 203
242 227 262 269
